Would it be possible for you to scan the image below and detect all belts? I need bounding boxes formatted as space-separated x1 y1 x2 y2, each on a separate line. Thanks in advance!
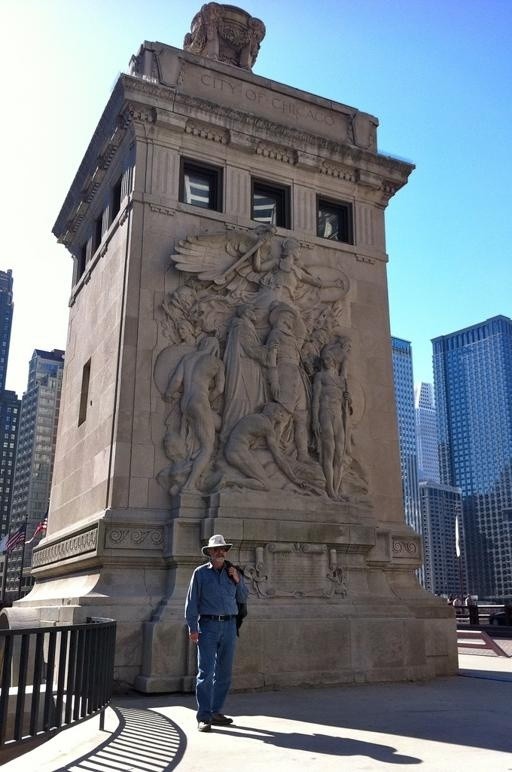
200 613 238 622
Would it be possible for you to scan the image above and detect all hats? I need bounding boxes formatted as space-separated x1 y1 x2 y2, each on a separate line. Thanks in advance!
200 534 231 558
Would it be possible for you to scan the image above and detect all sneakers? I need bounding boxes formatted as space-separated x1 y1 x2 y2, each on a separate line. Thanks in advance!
211 712 234 724
196 719 212 732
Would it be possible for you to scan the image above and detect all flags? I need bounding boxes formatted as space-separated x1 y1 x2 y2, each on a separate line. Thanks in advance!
6 524 27 553
25 516 49 544
0 534 10 554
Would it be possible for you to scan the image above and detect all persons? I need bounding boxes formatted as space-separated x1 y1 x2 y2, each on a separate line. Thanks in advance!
253 230 341 313
464 594 474 614
162 298 356 503
447 596 453 606
183 534 249 730
452 594 462 613
